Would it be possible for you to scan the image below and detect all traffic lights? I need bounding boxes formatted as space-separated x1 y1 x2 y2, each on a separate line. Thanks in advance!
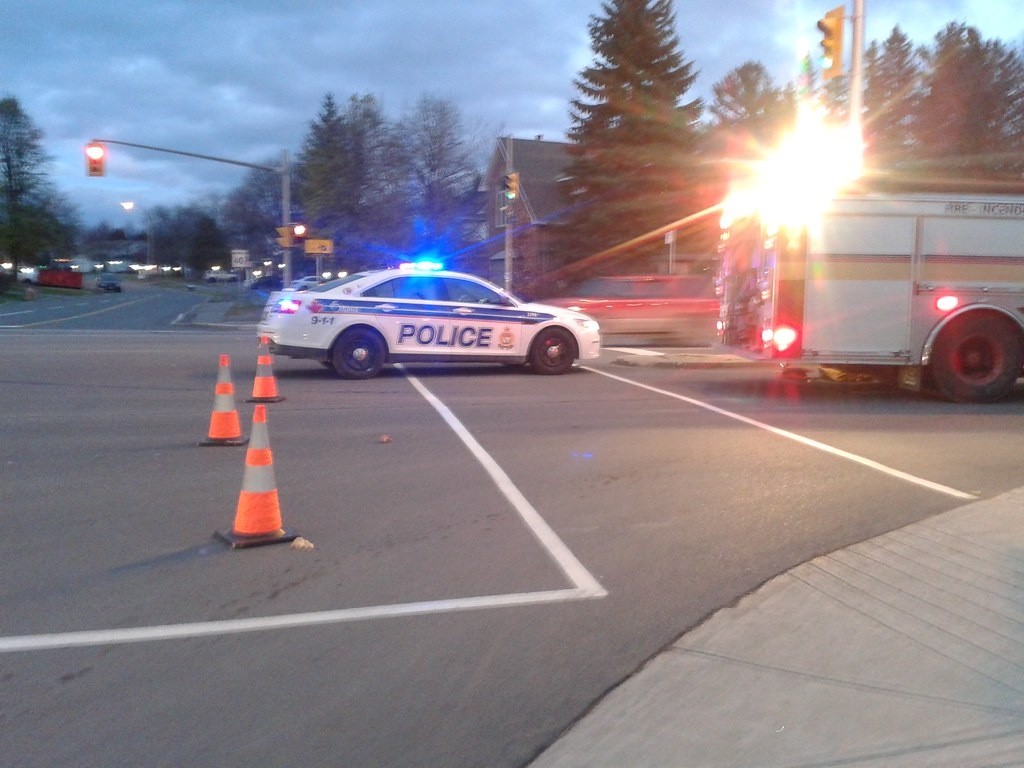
86 143 105 176
275 223 307 249
503 171 519 201
816 4 845 81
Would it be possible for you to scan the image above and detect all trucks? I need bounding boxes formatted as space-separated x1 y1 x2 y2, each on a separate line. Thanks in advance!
714 183 1024 403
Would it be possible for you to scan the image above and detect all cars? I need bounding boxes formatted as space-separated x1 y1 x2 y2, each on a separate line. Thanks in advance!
291 276 332 292
0 254 191 295
529 273 721 351
243 276 288 292
202 268 238 283
255 259 602 380
